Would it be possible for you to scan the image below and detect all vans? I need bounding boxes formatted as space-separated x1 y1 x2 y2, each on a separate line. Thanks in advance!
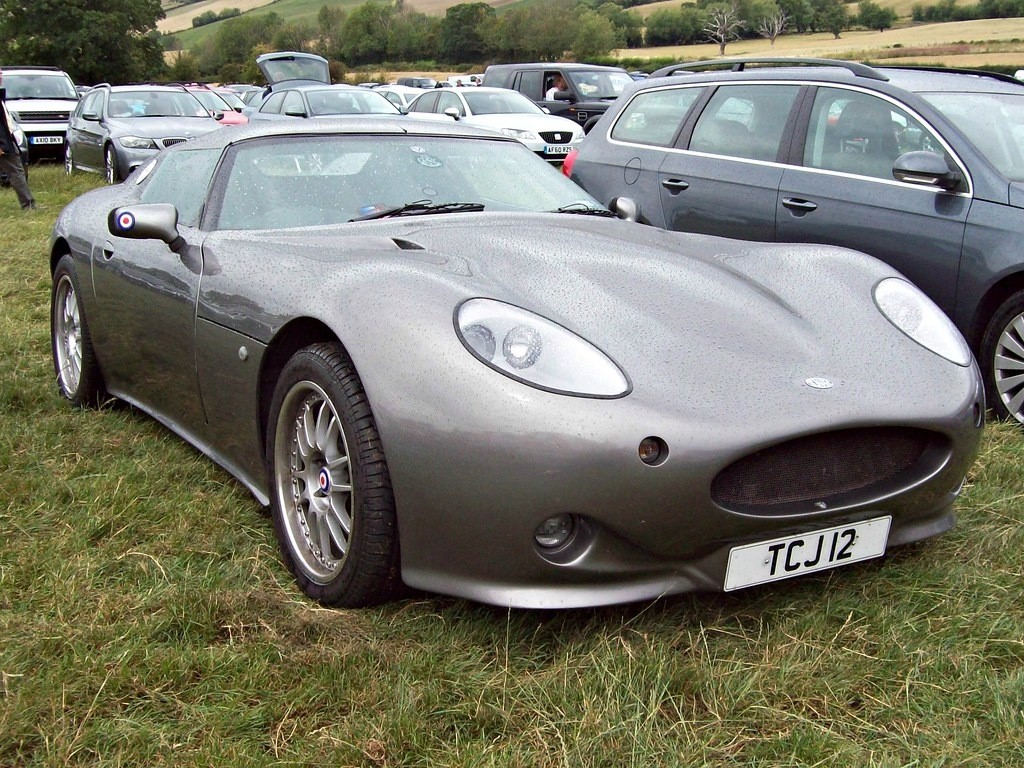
1 65 81 167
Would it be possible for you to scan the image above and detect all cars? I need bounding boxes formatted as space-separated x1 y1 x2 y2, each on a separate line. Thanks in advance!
627 71 940 161
248 85 408 172
403 87 586 169
0 114 29 186
76 51 485 127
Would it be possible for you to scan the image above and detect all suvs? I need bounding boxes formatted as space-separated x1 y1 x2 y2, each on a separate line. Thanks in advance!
63 82 224 186
482 63 692 140
560 55 1024 436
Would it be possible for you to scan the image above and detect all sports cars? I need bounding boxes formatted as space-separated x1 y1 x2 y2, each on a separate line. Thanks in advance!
49 117 987 609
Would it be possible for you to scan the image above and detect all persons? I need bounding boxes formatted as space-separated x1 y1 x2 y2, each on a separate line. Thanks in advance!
476 78 481 86
545 77 599 101
435 81 443 88
0 71 36 210
456 79 464 87
